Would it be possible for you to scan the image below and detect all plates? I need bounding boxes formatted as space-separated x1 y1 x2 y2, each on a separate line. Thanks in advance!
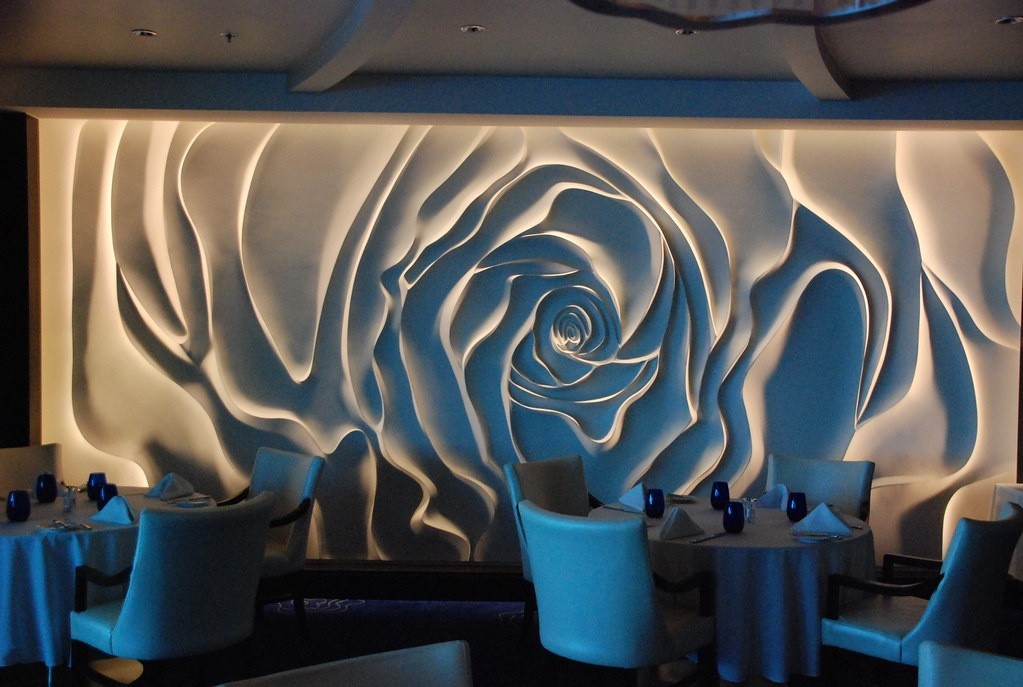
37 522 79 532
664 496 696 502
807 507 839 512
57 486 86 492
790 532 837 544
175 498 208 507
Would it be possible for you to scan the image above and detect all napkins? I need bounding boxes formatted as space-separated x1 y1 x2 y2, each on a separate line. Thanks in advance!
619 483 650 513
754 483 789 512
145 473 194 500
789 502 855 537
90 495 137 526
657 506 705 540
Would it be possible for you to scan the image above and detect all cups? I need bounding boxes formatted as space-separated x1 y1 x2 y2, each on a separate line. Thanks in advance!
97 484 118 511
87 473 106 500
723 501 745 534
787 492 807 523
6 490 31 522
36 474 57 503
645 489 665 518
710 481 729 509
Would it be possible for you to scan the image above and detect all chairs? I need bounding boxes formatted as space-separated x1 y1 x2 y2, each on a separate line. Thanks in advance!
70 491 274 687
820 499 1023 687
0 443 63 499
213 640 475 687
215 446 325 630
504 451 716 687
765 454 876 522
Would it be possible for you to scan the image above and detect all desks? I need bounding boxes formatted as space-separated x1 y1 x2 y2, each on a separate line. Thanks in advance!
585 496 876 683
0 486 217 687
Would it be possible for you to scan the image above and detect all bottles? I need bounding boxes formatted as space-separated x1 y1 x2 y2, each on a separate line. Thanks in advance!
742 498 754 522
63 486 77 512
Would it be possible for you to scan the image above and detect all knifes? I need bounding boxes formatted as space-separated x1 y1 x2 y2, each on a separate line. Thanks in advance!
603 505 646 514
690 532 726 543
